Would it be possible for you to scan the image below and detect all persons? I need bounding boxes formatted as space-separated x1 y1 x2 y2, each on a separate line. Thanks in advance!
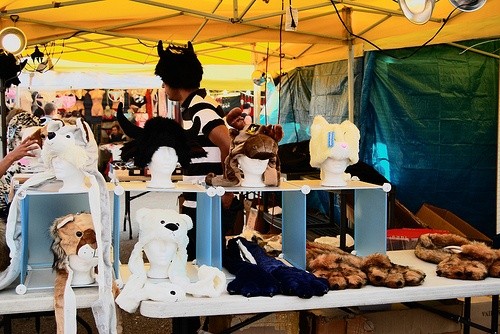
39 102 57 125
240 103 253 124
107 125 127 144
153 39 243 263
0 136 39 178
148 146 179 188
237 155 348 188
54 158 90 193
70 253 95 286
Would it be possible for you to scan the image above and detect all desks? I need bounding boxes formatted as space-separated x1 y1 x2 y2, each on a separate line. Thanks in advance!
119 249 500 334
115 170 183 240
0 274 99 334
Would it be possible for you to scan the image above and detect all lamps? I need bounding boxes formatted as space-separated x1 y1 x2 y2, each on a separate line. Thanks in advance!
449 0 486 12
398 0 439 25
0 27 27 56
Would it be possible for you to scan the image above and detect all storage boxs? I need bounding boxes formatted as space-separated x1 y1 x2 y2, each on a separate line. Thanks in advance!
416 204 493 240
208 294 493 333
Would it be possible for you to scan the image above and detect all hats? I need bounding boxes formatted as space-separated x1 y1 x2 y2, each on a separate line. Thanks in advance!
205 122 284 188
0 49 28 86
310 115 360 168
46 213 123 334
116 102 208 167
155 40 203 89
115 208 193 314
0 112 40 210
0 116 116 334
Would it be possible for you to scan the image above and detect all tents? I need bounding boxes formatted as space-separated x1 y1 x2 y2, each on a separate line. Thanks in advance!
0 0 500 334
